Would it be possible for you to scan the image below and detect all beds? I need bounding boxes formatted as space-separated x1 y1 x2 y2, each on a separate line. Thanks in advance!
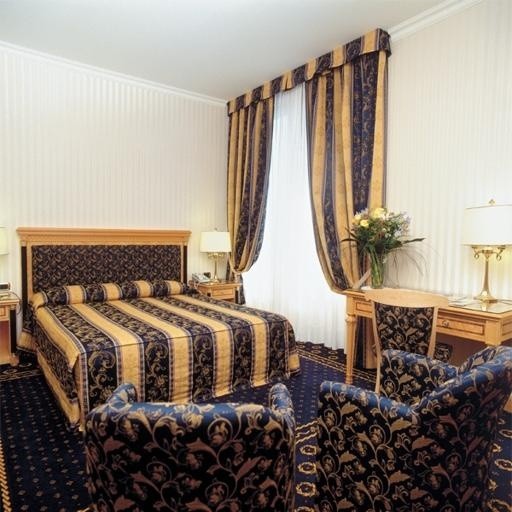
14 225 302 433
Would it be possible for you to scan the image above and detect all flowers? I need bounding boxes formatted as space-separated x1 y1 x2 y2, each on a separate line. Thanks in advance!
338 205 430 287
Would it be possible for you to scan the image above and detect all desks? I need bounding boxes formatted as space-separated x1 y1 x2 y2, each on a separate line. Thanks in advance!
341 285 512 385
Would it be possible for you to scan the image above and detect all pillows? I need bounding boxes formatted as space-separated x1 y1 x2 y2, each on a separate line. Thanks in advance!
43 282 121 302
124 279 187 296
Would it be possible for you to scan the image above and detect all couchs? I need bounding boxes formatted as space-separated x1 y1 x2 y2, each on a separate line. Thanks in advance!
313 343 512 512
79 381 300 511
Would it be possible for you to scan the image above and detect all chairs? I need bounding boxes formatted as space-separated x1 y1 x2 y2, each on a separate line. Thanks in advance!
367 300 455 394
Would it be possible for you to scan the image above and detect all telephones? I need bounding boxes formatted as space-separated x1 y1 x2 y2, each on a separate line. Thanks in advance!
192 273 210 283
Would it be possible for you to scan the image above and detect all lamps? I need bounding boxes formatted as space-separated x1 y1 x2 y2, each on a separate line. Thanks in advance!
0 226 13 298
458 199 512 305
196 226 232 285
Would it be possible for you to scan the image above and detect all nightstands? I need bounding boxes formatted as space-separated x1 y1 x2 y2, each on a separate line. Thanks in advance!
189 279 243 304
0 292 21 367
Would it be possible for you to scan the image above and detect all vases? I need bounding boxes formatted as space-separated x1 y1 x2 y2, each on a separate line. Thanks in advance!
368 252 388 289
